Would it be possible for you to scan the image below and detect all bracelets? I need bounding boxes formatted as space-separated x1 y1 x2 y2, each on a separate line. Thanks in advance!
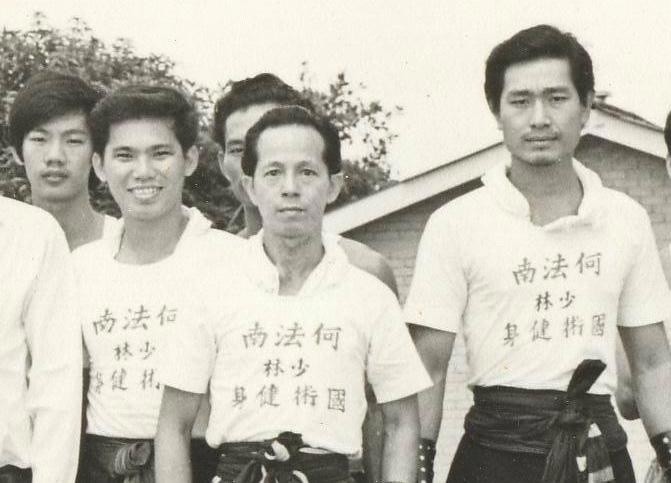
417 438 438 483
650 426 671 483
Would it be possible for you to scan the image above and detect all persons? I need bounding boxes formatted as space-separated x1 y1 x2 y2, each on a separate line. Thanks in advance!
69 84 252 482
400 22 671 483
10 69 121 253
216 75 399 481
2 195 84 483
151 105 434 482
614 111 671 482
